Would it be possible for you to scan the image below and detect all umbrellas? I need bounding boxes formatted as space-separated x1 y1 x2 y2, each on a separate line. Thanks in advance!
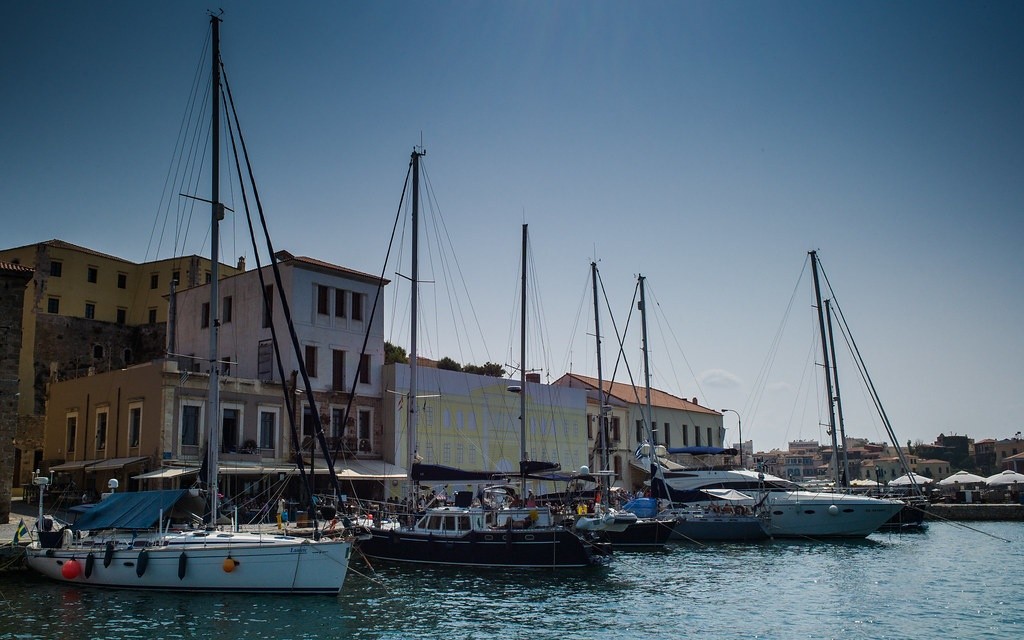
937 470 987 485
888 472 933 485
986 470 1024 485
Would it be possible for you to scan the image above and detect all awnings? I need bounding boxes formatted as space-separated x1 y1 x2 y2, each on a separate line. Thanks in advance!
169 457 408 481
49 460 103 471
85 456 150 471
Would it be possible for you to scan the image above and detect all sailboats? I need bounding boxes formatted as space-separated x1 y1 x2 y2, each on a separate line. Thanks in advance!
25 7 929 594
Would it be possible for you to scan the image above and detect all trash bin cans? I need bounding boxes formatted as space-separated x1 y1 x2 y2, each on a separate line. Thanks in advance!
297 511 308 528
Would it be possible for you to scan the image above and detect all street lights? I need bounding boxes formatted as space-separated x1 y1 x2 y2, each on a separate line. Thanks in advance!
721 409 743 470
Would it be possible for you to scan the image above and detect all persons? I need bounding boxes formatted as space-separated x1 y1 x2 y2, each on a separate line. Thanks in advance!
610 488 650 507
276 494 290 527
82 491 88 504
227 494 269 524
528 495 535 508
509 495 525 508
307 493 440 520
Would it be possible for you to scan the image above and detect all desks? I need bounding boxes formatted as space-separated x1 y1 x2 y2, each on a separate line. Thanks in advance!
20 484 33 503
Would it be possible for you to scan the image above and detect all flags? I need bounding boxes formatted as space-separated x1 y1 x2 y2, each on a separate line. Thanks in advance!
14 520 28 542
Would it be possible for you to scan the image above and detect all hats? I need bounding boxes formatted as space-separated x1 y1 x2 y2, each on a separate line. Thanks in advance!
528 489 532 491
